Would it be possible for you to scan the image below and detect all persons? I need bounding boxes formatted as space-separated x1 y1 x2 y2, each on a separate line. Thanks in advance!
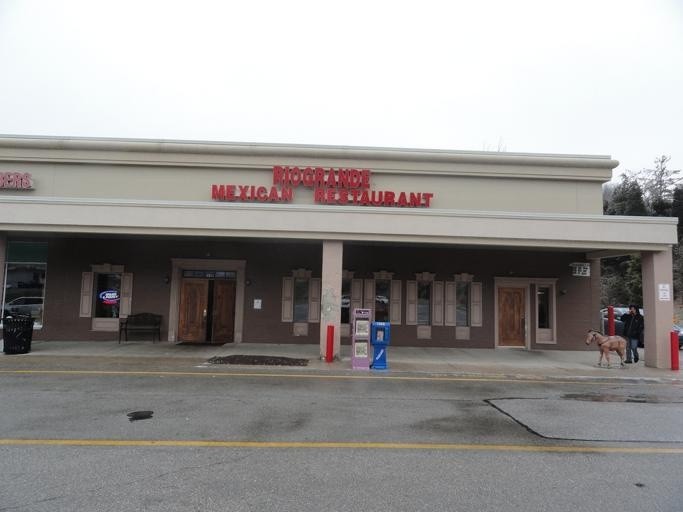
620 304 644 364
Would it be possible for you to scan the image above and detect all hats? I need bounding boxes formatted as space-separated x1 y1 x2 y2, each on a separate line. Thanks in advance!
629 303 639 314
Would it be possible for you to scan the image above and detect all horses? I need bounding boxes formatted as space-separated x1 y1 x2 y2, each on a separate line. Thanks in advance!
585 328 630 367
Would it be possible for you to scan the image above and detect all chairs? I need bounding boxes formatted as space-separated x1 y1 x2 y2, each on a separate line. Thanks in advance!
119 313 163 345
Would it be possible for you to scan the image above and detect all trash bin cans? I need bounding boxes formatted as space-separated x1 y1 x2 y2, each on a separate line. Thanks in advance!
3 318 36 354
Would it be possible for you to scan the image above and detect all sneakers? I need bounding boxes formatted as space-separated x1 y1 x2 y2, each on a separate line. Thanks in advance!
623 353 638 362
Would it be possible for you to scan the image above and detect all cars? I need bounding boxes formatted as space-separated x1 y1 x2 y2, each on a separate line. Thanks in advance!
600 307 683 350
4 296 44 316
376 296 389 304
342 295 350 308
